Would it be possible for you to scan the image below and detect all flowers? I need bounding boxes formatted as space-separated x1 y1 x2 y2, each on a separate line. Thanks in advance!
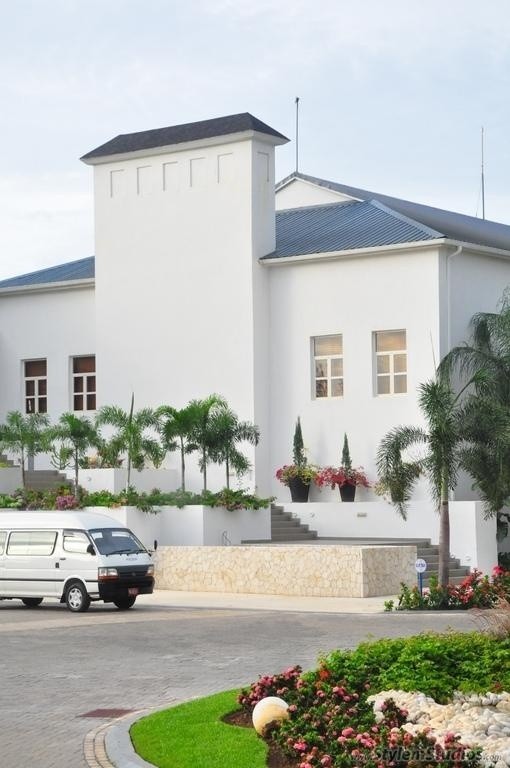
316 465 373 489
272 465 325 485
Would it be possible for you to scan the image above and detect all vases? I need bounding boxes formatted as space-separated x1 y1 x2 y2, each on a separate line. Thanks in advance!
289 484 310 502
338 483 356 502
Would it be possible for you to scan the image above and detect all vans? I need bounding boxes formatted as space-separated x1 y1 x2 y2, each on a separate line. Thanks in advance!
0 510 158 612
